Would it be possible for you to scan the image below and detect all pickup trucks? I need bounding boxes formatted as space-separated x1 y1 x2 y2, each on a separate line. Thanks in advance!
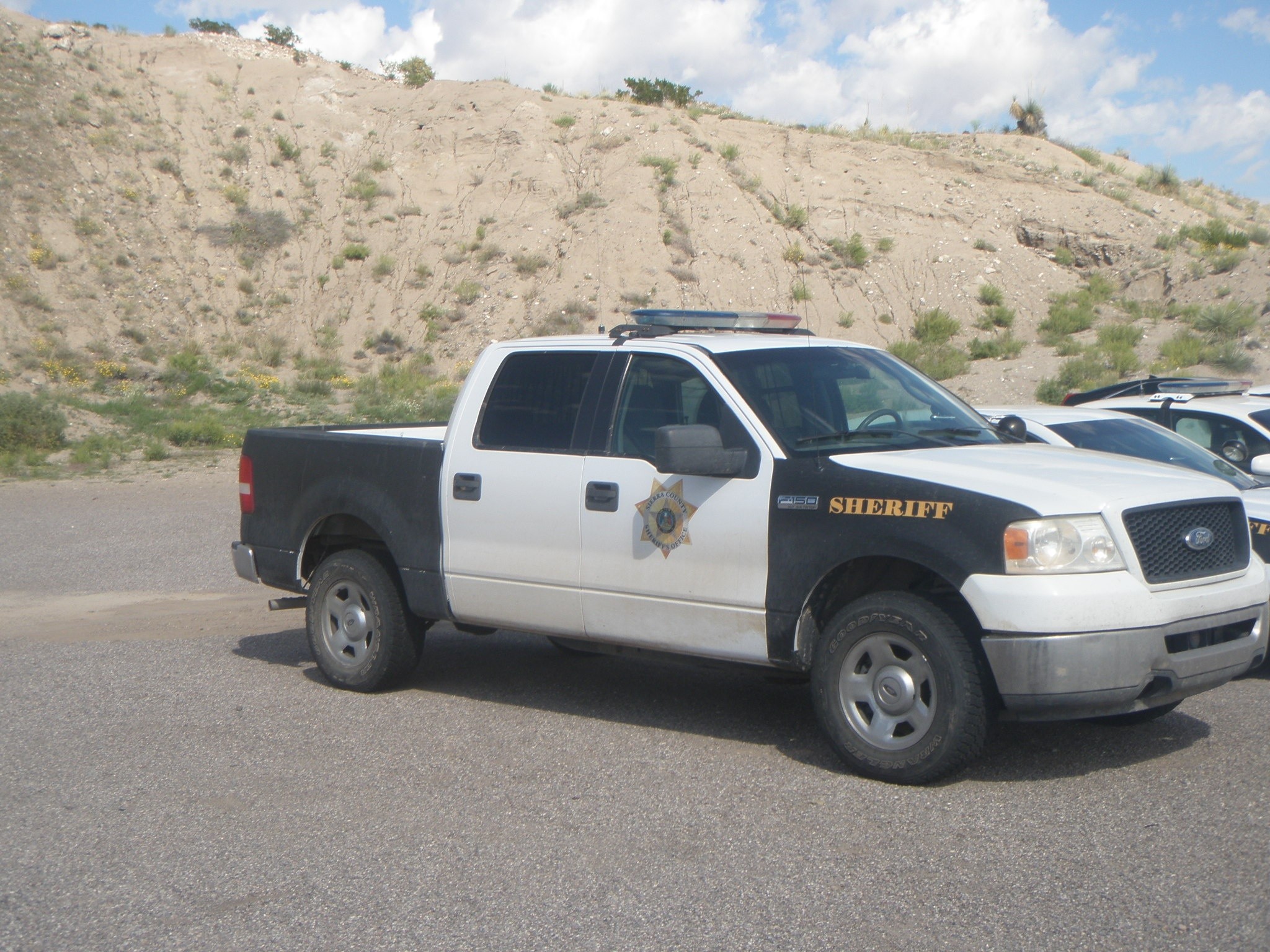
228 307 1269 785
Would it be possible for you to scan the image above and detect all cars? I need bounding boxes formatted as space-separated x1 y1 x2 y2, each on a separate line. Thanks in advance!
1074 378 1269 486
1059 374 1207 406
845 405 1270 574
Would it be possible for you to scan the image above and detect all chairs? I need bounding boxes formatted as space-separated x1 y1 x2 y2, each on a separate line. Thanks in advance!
1209 422 1238 452
622 384 681 458
698 385 777 443
